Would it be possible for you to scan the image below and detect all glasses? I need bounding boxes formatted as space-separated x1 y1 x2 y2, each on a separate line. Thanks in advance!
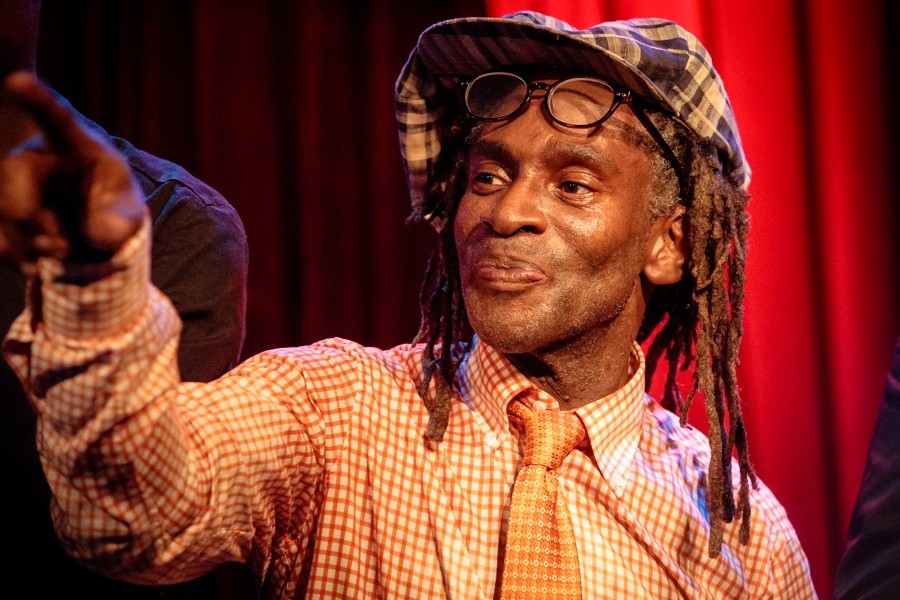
456 70 665 128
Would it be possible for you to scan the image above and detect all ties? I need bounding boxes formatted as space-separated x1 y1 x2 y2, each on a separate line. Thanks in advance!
499 398 591 600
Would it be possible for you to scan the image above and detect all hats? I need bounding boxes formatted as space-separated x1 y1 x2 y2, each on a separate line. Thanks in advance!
394 10 751 238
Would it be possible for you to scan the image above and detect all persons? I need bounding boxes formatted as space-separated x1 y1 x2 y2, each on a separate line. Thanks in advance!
0 0 819 600
831 339 900 600
